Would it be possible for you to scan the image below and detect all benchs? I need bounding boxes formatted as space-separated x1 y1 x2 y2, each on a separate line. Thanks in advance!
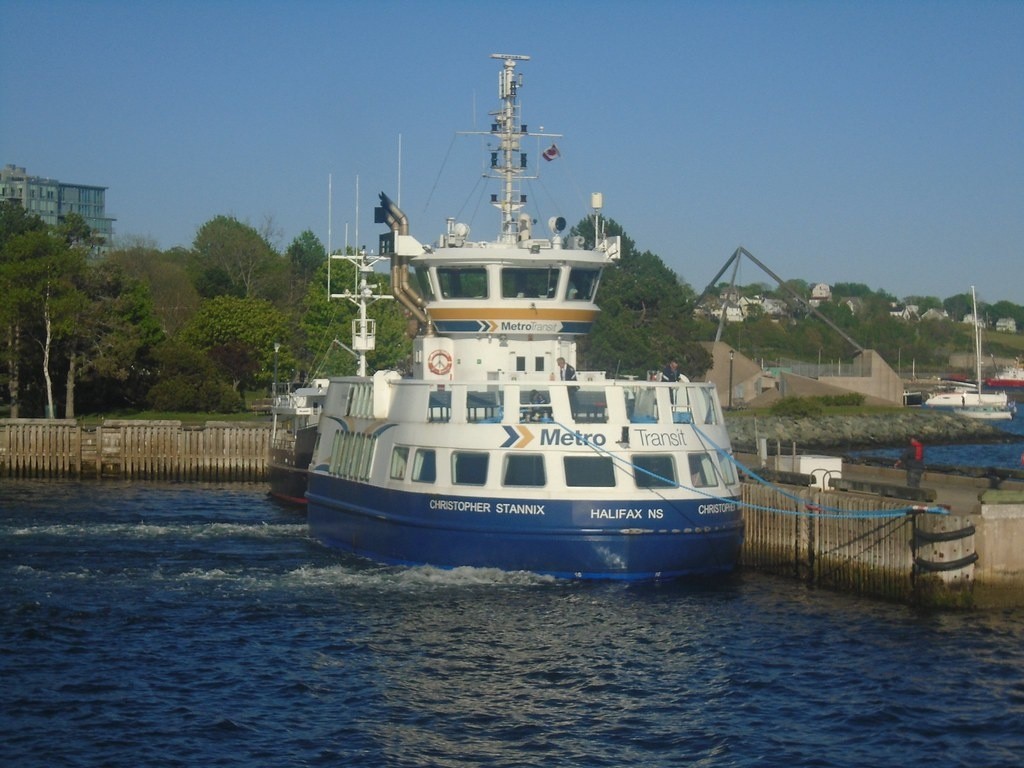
520 389 607 422
428 391 501 420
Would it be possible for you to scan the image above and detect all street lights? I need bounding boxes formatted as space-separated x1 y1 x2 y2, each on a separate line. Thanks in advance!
273 338 279 422
728 350 734 410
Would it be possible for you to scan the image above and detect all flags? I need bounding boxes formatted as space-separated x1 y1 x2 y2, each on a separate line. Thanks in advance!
542 144 561 161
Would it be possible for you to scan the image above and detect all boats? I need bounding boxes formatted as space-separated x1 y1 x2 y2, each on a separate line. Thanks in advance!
921 323 1008 407
267 53 745 578
985 356 1024 391
954 286 1013 421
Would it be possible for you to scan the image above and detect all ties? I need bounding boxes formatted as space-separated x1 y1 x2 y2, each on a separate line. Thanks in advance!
560 369 562 381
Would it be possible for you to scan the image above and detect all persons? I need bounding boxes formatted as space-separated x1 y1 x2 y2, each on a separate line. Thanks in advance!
528 390 552 421
893 430 926 499
656 359 681 419
556 357 581 419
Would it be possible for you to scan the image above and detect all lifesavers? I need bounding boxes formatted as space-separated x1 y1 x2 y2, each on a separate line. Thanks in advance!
428 351 452 374
